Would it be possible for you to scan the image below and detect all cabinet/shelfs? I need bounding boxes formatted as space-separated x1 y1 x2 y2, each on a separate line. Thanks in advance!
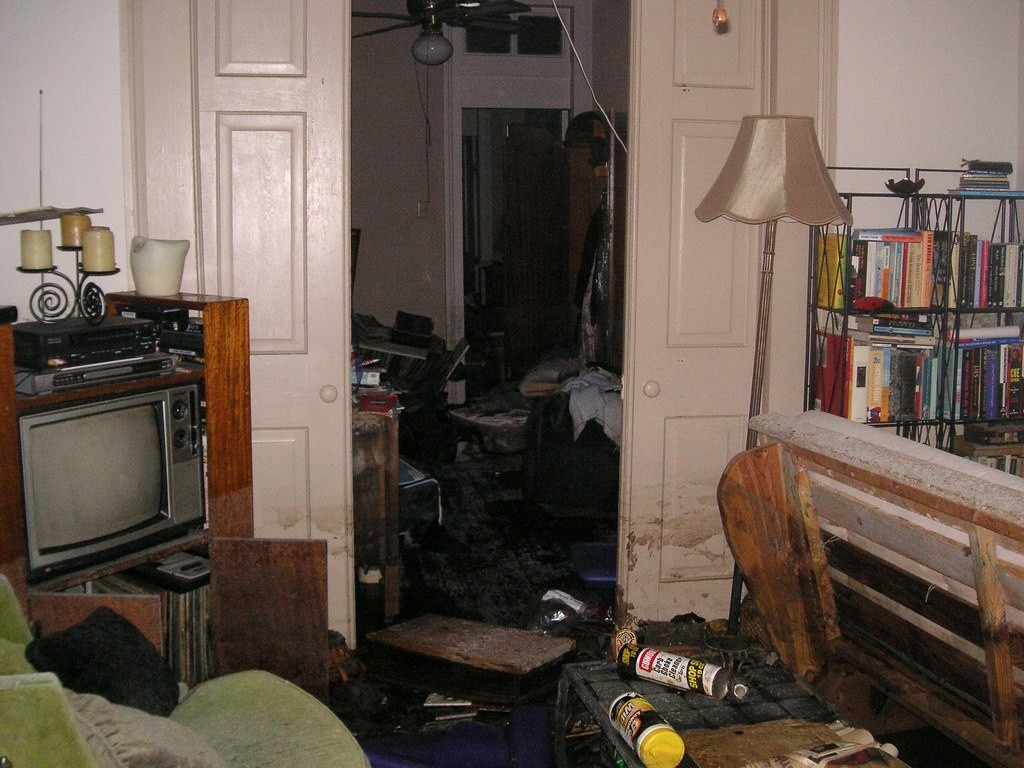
352 412 399 621
103 292 255 683
810 166 1023 478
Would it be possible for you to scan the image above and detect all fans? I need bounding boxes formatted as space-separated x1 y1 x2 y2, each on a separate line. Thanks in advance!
350 0 533 34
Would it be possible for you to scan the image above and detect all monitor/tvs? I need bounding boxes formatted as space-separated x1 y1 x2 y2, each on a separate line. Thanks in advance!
18 384 207 584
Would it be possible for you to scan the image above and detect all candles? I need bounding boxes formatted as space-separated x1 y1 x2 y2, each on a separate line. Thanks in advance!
81 229 115 273
60 212 91 247
19 229 53 269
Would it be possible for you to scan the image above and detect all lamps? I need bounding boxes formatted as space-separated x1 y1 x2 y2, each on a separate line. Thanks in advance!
692 113 854 656
411 23 454 66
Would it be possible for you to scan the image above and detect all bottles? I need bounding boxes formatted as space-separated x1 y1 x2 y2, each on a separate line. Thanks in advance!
608 687 685 768
616 641 748 701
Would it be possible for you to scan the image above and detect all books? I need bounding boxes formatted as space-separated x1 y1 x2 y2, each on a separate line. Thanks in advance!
352 331 469 412
816 159 1024 474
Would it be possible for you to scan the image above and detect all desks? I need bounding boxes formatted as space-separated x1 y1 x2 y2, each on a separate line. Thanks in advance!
555 657 906 767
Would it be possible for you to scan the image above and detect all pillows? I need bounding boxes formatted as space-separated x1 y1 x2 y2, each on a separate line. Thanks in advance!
67 694 230 768
23 603 182 720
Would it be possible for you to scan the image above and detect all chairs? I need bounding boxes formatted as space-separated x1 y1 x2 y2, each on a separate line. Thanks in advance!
2 574 373 768
